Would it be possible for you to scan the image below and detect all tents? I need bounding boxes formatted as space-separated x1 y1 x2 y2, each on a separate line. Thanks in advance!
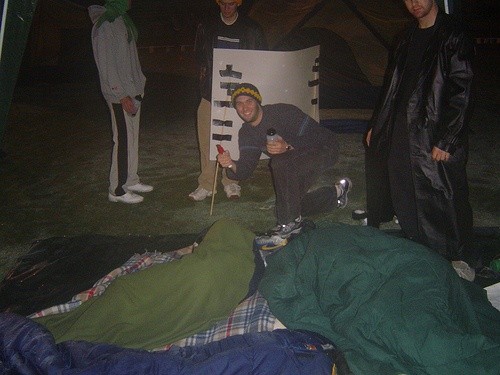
0 0 40 142
249 0 462 132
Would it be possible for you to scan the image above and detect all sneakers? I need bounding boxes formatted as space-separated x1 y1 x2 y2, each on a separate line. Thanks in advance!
223 182 241 199
274 216 302 238
336 177 351 209
188 186 217 201
109 192 144 203
127 183 153 192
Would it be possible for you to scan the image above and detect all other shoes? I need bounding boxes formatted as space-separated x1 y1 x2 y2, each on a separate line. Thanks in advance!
452 260 476 281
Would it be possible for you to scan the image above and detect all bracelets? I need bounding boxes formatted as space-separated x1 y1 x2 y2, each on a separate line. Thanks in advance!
285 145 291 151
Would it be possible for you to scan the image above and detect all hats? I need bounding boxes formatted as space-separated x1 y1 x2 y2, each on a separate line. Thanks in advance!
231 83 261 104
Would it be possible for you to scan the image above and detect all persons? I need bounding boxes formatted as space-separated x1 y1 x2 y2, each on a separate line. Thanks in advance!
188 0 267 201
217 83 352 239
88 1 153 202
366 0 475 280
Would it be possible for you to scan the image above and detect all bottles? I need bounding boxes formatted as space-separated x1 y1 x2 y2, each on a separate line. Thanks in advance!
266 128 279 142
127 94 143 118
